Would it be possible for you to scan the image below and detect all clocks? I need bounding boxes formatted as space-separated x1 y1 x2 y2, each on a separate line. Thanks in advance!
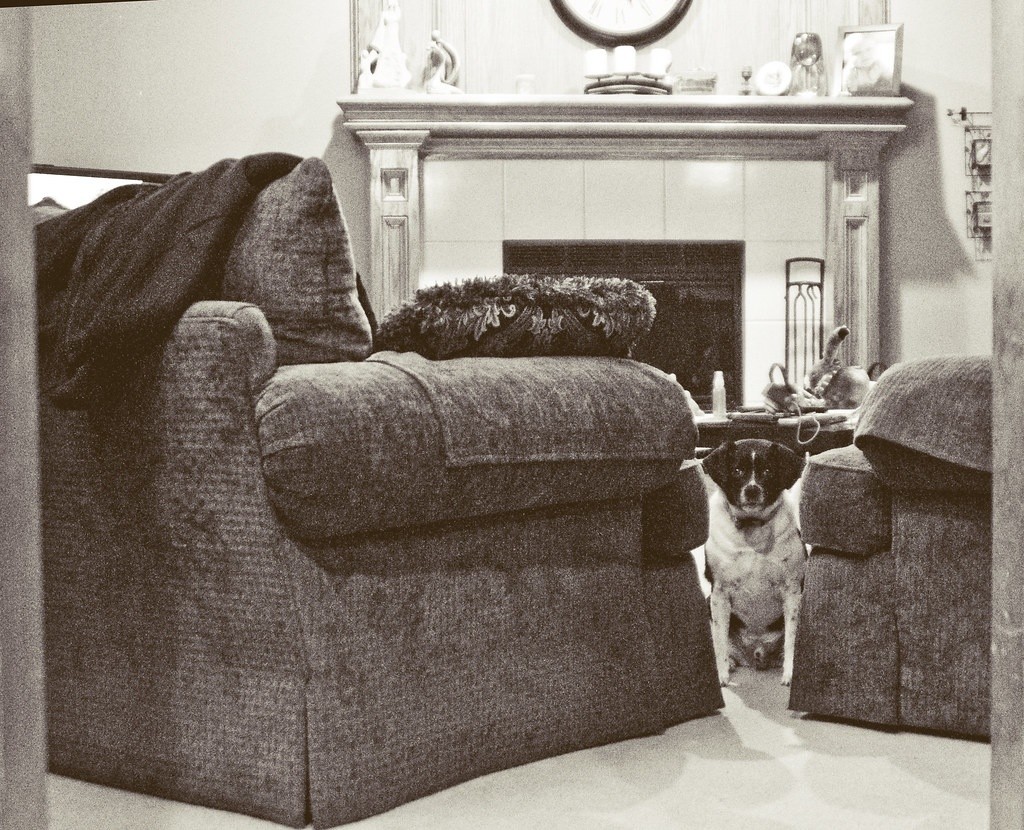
551 0 693 52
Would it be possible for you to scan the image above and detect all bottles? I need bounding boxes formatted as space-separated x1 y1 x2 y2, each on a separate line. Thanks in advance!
712 371 727 421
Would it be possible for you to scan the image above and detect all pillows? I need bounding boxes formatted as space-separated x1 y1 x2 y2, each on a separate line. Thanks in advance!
374 273 657 357
28 160 373 366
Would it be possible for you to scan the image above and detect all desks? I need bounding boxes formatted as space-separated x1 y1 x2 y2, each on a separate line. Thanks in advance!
696 412 856 453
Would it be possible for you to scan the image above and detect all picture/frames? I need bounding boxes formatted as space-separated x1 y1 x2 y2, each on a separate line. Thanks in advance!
836 23 905 95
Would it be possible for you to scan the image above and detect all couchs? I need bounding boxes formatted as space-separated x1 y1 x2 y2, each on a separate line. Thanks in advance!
38 300 725 830
785 355 991 740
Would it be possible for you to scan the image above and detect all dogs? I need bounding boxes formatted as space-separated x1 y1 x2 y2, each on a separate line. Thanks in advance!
698 437 809 691
